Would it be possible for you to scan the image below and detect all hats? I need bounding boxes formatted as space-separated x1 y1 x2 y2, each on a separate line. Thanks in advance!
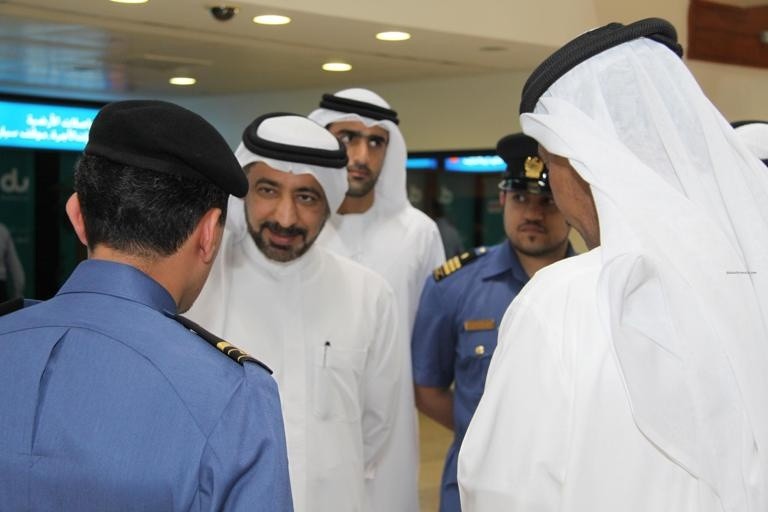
84 99 250 199
496 132 549 199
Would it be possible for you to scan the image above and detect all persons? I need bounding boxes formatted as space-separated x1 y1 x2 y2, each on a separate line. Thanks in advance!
456 15 767 512
181 108 421 511
303 87 445 348
409 129 583 512
1 99 296 510
725 117 768 164
1 225 27 300
